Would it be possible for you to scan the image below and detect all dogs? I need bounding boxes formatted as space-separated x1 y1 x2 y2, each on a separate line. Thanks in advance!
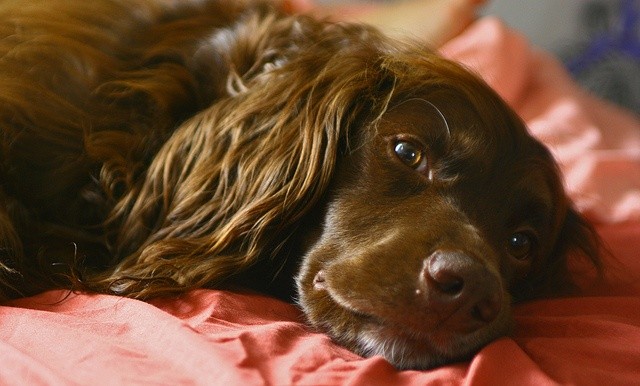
0 0 630 371
